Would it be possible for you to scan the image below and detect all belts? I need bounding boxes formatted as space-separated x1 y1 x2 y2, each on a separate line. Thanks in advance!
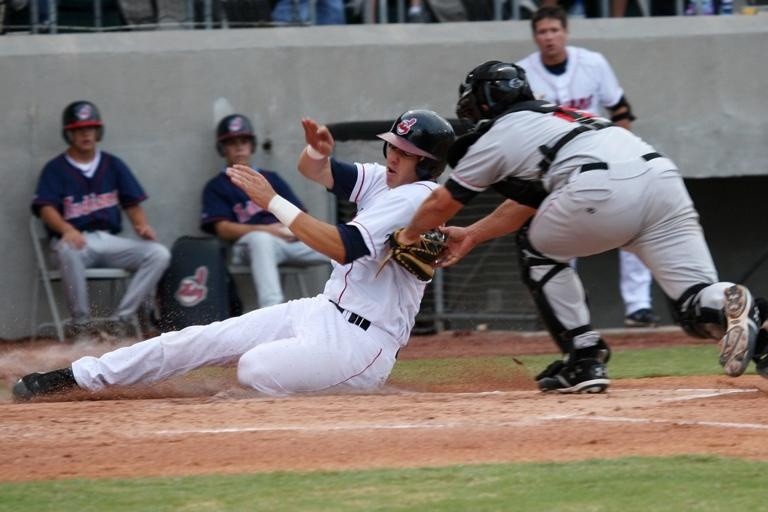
579 151 660 170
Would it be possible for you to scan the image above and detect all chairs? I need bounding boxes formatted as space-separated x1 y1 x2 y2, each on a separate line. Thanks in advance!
227 262 309 298
28 214 157 343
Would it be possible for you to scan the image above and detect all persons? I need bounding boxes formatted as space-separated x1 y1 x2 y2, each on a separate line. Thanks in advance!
1 0 768 33
30 102 174 344
199 113 334 307
386 61 767 395
510 4 664 328
11 110 456 401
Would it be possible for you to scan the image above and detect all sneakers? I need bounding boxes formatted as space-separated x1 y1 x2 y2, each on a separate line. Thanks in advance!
717 284 759 378
71 322 126 346
535 357 610 393
14 367 74 397
623 311 660 328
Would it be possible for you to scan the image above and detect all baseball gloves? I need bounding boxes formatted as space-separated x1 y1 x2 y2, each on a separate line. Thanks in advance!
376 229 446 283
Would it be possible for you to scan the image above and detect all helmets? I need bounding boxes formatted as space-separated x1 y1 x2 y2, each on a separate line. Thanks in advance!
457 59 533 125
216 115 257 155
62 100 103 143
376 106 455 178
